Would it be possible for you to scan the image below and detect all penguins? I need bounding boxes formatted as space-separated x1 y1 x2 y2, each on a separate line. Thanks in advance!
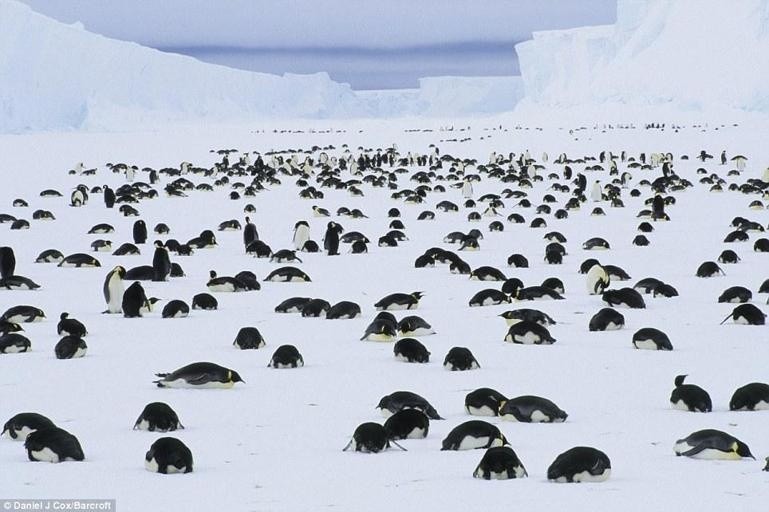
0 144 769 485
